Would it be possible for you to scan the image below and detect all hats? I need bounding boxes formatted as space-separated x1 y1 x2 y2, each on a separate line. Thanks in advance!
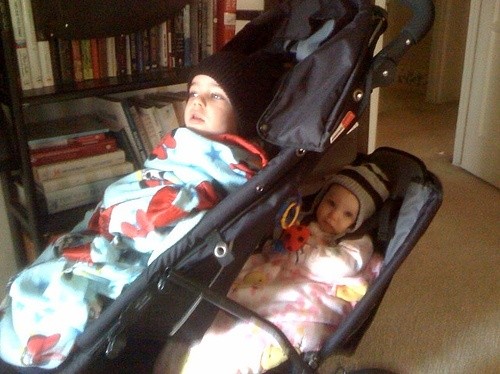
311 163 392 246
186 51 256 139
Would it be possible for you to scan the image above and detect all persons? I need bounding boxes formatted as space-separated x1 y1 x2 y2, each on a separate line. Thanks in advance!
155 162 392 373
0 30 289 373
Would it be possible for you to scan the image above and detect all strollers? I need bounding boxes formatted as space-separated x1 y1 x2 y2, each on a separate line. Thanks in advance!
0 0 448 374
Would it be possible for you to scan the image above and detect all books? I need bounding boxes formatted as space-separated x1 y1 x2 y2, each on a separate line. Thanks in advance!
14 87 190 216
2 0 239 92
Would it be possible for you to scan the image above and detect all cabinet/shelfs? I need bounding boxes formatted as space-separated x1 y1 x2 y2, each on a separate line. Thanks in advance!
0 0 369 270
